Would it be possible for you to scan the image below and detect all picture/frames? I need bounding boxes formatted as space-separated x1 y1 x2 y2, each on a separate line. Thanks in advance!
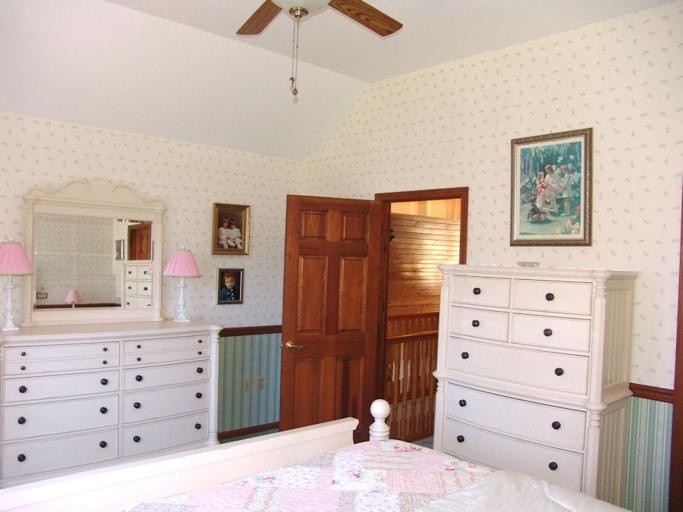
510 127 592 246
115 239 124 261
216 268 243 304
211 202 250 255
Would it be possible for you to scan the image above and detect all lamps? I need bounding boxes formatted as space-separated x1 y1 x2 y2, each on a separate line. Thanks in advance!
162 249 201 323
0 242 37 331
64 289 82 309
271 0 333 96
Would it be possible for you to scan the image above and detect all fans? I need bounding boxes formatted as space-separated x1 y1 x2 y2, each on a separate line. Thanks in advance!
236 0 404 38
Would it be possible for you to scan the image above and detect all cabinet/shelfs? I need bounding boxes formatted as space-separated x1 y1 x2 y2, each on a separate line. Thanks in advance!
430 264 638 507
0 320 223 490
120 260 154 309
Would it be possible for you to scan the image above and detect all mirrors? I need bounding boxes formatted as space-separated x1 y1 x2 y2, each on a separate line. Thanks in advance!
21 180 167 328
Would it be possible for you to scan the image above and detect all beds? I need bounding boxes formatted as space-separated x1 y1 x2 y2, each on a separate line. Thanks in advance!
0 399 634 512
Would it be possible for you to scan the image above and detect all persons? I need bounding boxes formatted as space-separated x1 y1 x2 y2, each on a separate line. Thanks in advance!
219 271 240 300
533 164 570 216
218 216 242 248
